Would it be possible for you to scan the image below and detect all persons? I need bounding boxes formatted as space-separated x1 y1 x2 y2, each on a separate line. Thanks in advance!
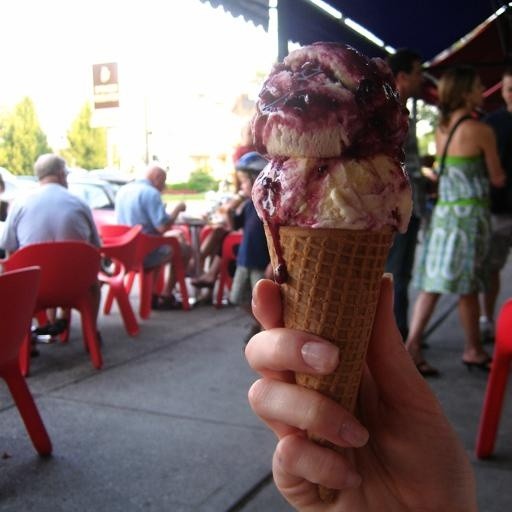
190 152 276 346
384 50 425 341
0 154 103 333
115 168 192 308
406 65 506 375
244 272 474 512
0 174 10 258
478 67 512 342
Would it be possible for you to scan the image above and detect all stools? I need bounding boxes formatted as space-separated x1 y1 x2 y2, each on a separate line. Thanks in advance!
476 296 512 460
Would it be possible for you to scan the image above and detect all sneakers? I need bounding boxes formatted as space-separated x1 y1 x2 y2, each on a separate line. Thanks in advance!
36 324 61 336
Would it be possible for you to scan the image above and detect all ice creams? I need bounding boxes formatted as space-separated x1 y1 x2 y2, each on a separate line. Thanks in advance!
251 40 415 503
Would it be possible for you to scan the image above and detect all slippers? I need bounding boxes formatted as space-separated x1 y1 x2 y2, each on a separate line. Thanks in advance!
463 353 492 371
417 361 439 376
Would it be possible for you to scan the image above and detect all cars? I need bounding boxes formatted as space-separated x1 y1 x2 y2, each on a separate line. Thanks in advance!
0 165 139 238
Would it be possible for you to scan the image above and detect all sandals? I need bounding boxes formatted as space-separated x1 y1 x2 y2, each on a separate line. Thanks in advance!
157 294 182 310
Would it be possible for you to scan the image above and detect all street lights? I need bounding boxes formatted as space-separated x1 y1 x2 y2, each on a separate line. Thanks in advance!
145 130 153 165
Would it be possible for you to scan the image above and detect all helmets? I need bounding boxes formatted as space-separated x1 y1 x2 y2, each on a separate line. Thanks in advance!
236 151 269 171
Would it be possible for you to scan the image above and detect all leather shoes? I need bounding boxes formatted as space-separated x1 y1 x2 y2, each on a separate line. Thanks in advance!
191 278 214 288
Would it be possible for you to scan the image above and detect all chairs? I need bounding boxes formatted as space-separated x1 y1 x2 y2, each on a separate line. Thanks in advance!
1 215 142 460
94 218 253 320
